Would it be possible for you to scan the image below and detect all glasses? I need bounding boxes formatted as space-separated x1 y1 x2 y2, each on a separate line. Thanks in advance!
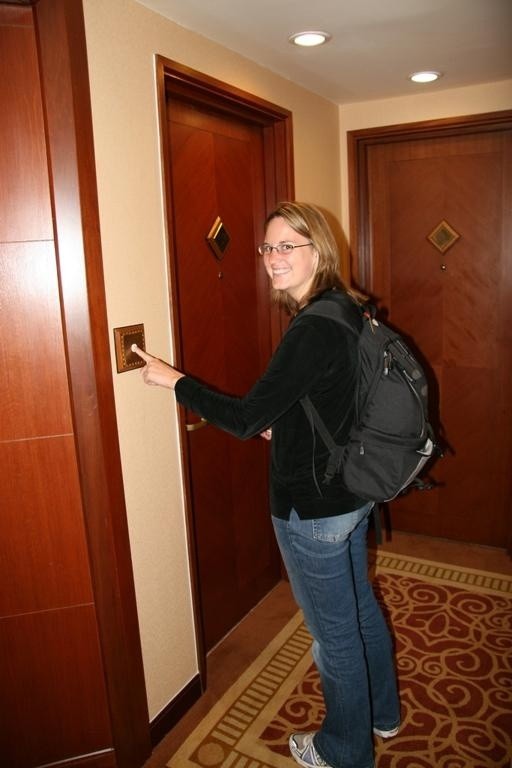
258 243 315 255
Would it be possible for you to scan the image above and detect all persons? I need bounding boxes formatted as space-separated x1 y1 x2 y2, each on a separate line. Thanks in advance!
134 202 404 768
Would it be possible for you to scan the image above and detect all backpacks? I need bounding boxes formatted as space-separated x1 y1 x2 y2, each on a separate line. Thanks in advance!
282 300 444 503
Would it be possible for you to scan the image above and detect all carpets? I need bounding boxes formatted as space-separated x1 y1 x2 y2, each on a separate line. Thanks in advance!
164 546 512 768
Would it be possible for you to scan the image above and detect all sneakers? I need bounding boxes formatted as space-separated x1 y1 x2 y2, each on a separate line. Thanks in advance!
373 726 399 738
288 733 332 767
